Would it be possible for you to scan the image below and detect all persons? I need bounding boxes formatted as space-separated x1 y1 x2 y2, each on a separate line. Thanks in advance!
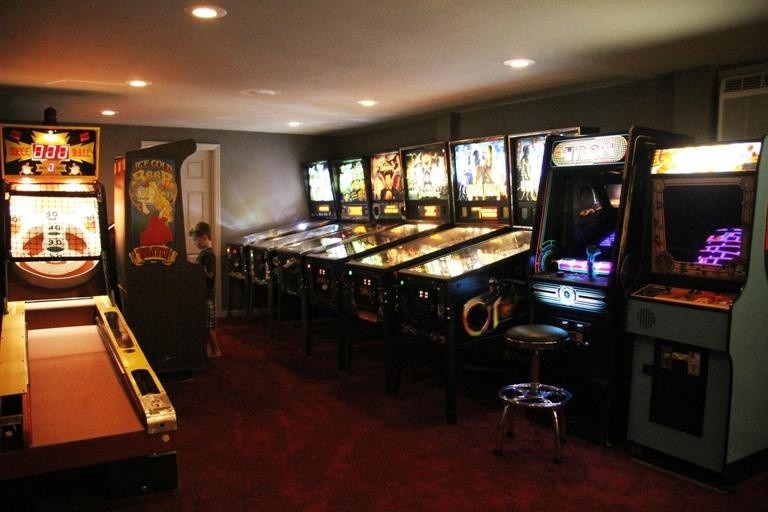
520 144 533 201
190 221 222 359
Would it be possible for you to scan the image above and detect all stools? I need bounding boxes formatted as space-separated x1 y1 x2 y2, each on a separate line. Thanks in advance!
494 322 574 466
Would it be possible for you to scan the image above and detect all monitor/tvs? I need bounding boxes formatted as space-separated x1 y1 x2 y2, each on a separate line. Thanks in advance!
7 191 103 261
540 168 624 277
649 177 757 285
